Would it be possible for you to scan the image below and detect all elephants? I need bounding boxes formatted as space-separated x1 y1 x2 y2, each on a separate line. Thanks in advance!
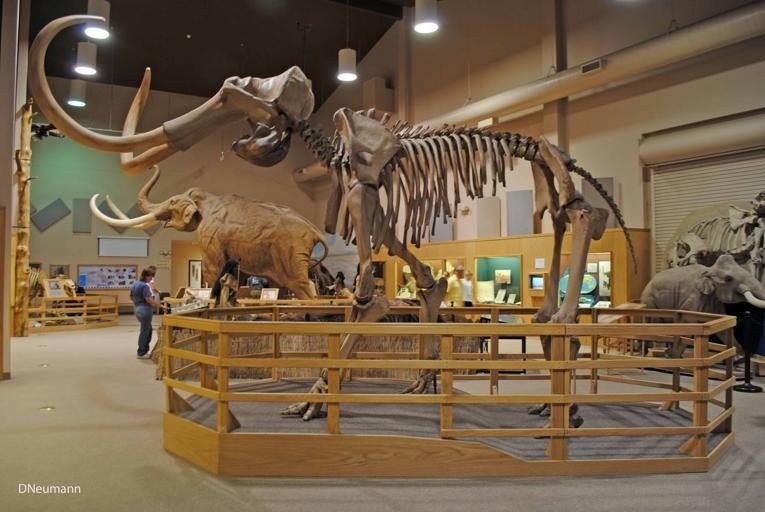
639 251 765 357
89 165 331 306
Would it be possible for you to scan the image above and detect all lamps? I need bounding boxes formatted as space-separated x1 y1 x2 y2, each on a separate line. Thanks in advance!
66 0 112 108
335 0 358 82
414 0 439 34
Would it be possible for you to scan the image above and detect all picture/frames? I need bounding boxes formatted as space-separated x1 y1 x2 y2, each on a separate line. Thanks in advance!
28 262 138 291
188 259 203 289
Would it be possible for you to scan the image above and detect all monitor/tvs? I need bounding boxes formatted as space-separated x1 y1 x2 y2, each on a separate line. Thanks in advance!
259 288 280 301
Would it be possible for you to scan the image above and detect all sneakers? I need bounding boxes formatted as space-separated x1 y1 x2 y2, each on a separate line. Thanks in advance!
137 350 151 359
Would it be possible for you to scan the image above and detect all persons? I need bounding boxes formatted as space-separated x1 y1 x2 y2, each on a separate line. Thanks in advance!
128 268 169 360
446 266 463 308
460 271 474 306
211 257 240 308
146 264 160 297
